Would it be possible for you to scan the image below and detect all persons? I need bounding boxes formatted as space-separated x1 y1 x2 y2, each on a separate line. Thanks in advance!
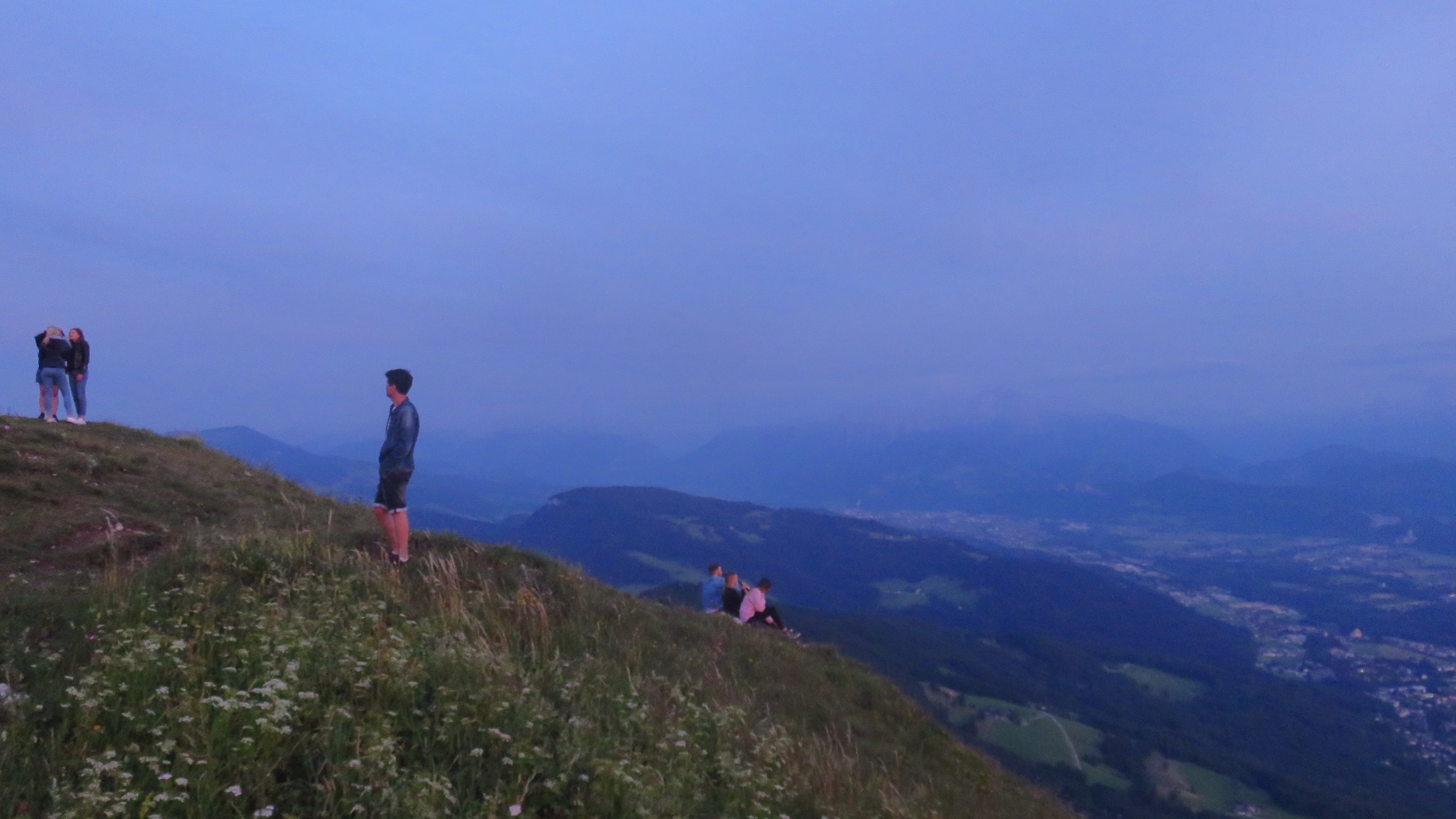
373 369 420 565
34 326 90 425
701 563 801 639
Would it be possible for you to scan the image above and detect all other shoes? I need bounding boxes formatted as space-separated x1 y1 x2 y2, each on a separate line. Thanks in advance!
76 417 89 425
37 413 59 422
67 417 79 424
389 552 409 568
